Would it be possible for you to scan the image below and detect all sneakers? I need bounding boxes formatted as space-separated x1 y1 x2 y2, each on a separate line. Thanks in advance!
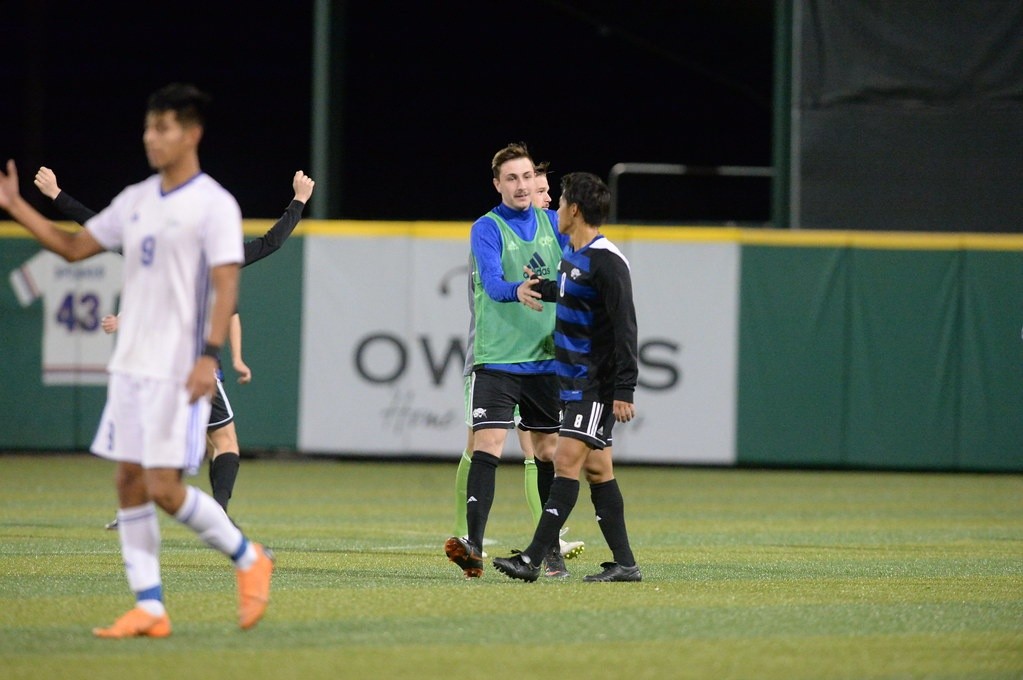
445 537 483 578
559 527 585 559
238 542 274 629
583 562 641 582
493 550 540 583
93 607 170 637
543 544 570 579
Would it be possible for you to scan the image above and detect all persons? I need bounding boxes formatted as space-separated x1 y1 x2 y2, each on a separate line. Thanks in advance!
0 82 273 638
35 166 321 529
494 173 643 582
455 145 586 560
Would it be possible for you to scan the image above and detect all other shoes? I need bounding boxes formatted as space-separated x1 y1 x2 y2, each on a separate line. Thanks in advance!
104 515 118 532
